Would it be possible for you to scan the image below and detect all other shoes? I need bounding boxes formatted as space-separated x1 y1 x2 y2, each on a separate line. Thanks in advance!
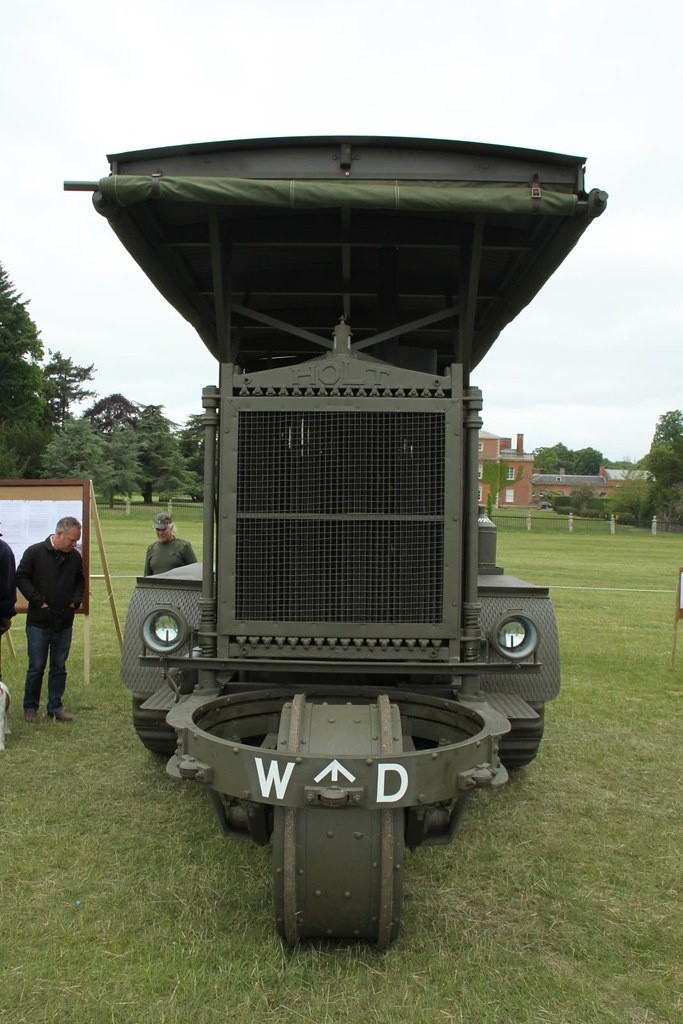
47 710 73 722
24 708 38 724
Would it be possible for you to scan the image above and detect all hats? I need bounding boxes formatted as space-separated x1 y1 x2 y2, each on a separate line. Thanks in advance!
153 513 173 530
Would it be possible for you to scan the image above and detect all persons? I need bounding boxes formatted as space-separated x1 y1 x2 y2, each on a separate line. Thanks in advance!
16 517 85 722
144 513 198 575
0 533 17 682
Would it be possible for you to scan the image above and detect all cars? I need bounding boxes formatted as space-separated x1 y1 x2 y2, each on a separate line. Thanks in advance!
542 501 552 510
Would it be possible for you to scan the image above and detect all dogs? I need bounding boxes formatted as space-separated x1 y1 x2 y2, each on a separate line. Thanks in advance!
0 680 12 751
